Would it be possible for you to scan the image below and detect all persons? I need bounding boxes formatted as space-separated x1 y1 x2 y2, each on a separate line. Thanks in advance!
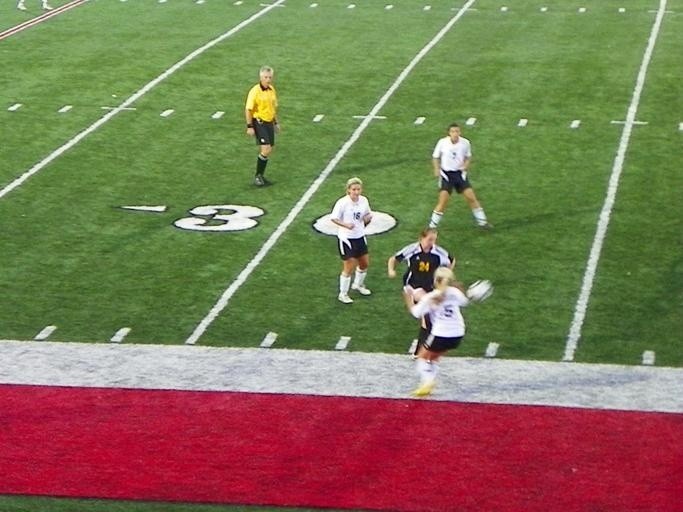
425 122 490 229
16 0 54 12
402 266 472 396
327 175 372 304
245 65 280 186
385 225 456 362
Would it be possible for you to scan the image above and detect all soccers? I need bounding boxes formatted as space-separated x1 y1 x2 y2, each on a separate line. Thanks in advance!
467 280 494 303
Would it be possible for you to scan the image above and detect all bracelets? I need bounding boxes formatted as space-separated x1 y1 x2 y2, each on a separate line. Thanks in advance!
246 121 253 127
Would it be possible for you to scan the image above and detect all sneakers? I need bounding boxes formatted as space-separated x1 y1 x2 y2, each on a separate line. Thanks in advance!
255 175 265 185
338 293 353 304
351 283 371 295
414 383 436 396
